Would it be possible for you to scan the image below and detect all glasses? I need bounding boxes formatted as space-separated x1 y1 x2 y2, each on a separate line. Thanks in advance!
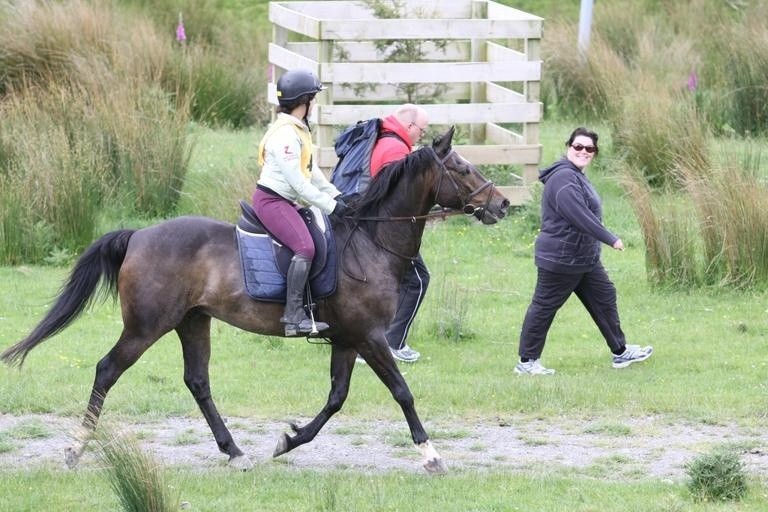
412 121 427 136
570 143 598 152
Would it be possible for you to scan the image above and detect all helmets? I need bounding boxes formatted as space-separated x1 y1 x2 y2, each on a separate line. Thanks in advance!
277 69 329 101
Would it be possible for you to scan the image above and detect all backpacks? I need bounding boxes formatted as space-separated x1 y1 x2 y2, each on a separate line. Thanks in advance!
329 117 405 196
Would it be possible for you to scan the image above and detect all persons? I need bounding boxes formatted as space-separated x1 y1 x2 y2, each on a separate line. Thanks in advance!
514 125 657 379
327 101 430 363
251 67 348 339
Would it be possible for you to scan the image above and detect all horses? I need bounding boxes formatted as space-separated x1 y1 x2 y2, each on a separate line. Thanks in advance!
0 124 511 478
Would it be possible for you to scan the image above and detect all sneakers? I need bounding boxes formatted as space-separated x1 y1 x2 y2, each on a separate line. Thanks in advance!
389 343 420 362
354 354 367 364
612 344 654 369
513 359 556 375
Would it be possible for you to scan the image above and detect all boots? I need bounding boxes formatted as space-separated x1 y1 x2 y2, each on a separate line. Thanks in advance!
281 255 331 333
